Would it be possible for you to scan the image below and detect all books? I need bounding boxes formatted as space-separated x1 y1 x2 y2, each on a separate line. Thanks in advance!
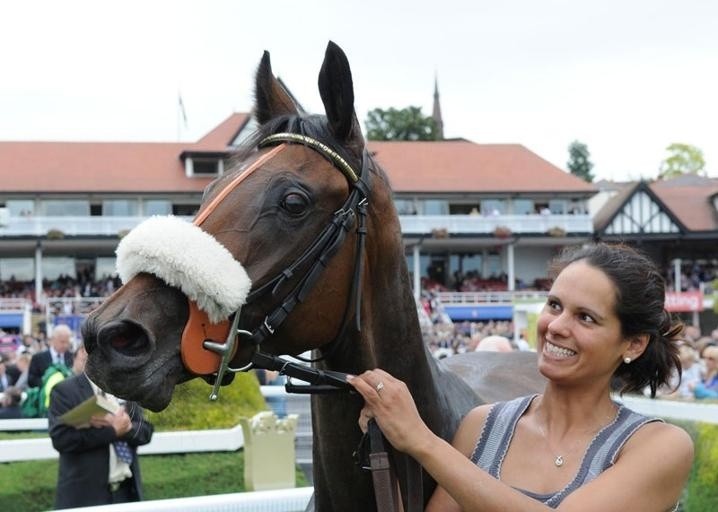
61 395 120 427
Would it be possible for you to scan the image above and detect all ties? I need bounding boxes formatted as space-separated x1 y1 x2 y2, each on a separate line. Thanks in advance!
112 440 133 466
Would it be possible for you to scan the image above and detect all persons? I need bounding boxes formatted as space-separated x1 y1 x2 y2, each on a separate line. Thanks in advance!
651 259 717 399
428 319 535 362
0 267 123 419
48 371 154 510
347 242 695 512
425 260 529 304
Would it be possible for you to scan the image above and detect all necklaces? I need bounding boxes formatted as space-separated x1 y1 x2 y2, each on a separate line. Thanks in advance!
533 414 582 466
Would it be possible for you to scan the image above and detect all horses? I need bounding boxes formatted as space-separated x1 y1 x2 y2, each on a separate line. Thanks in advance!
80 38 548 511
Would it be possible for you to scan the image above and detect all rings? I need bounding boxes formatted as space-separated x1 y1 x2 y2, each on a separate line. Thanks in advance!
376 383 384 392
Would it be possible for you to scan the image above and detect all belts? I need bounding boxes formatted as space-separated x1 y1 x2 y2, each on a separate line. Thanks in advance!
109 479 131 491
